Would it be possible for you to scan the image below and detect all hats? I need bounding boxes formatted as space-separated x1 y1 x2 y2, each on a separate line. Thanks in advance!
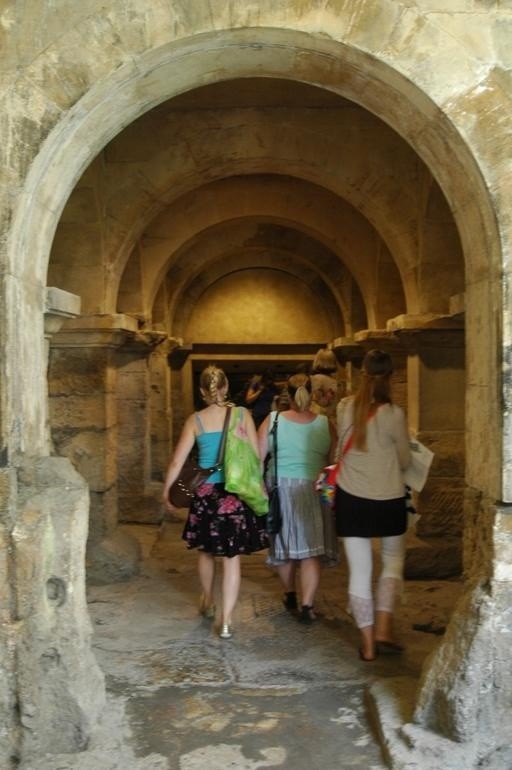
363 349 394 377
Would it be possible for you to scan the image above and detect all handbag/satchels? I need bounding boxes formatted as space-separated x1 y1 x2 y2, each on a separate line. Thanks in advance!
223 405 270 518
312 463 339 507
170 464 212 507
256 486 284 533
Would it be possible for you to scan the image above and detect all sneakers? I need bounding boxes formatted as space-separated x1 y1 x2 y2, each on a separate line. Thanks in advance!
282 591 299 611
199 593 216 619
220 625 233 639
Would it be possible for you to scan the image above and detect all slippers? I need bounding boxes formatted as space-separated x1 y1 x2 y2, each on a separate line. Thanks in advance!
357 636 406 662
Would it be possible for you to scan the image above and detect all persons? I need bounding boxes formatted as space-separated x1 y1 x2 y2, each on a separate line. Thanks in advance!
332 346 414 663
158 361 261 639
239 346 349 623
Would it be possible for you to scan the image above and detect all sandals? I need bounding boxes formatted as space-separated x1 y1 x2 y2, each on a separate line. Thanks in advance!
301 605 322 625
